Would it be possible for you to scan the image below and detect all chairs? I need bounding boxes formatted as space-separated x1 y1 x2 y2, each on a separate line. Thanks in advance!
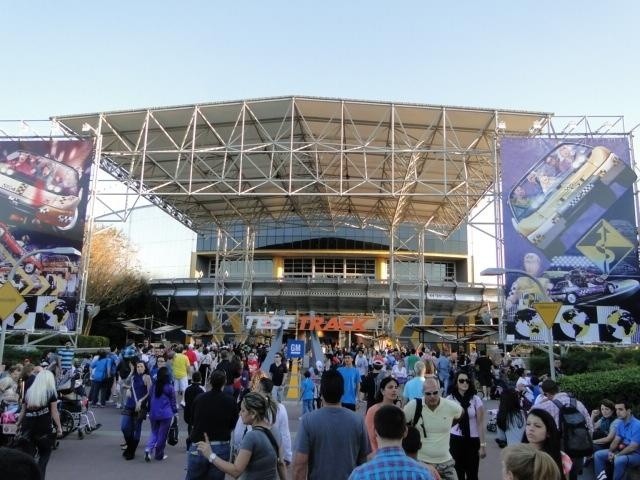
51 392 103 439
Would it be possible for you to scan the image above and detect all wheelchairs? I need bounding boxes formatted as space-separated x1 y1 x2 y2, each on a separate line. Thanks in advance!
53 399 103 440
82 372 91 388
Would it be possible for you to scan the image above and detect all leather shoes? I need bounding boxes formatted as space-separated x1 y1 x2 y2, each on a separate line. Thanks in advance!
143 448 152 463
155 454 169 461
120 439 128 449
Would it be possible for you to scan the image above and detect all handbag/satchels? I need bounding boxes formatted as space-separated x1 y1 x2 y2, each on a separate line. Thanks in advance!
136 405 147 419
167 416 181 447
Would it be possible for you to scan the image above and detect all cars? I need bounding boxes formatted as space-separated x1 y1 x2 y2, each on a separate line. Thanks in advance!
548 275 619 305
543 265 586 279
0 150 81 235
507 141 638 263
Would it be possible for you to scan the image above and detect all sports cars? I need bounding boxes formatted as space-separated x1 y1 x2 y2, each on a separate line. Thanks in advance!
0 221 42 275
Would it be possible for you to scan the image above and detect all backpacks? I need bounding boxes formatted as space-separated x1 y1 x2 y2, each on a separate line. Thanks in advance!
591 427 609 449
558 404 596 458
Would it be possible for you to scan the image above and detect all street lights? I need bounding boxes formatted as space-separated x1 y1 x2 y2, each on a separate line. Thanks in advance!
0 246 82 365
479 268 556 384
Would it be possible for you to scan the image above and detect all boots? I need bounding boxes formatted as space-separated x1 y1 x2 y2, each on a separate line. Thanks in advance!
122 434 141 460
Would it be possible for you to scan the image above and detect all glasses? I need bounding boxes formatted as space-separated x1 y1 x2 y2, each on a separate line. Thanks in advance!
274 356 281 358
424 390 439 396
457 378 470 384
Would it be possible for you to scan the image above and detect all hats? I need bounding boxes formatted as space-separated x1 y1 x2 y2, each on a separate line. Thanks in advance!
524 253 541 264
541 379 561 395
373 359 384 366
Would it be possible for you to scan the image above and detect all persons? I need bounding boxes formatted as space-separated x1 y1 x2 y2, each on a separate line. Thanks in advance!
505 252 552 314
511 141 578 214
12 151 66 192
1 341 639 480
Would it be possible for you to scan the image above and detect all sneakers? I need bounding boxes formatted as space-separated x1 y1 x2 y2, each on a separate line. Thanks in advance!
116 402 122 409
487 397 491 400
297 415 303 420
100 405 106 408
481 397 487 400
176 403 180 408
92 405 98 408
180 400 186 408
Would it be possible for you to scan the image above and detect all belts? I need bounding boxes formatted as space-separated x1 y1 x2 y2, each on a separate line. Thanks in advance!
191 440 230 447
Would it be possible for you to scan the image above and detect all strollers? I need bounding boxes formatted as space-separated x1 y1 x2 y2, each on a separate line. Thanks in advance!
486 408 498 432
